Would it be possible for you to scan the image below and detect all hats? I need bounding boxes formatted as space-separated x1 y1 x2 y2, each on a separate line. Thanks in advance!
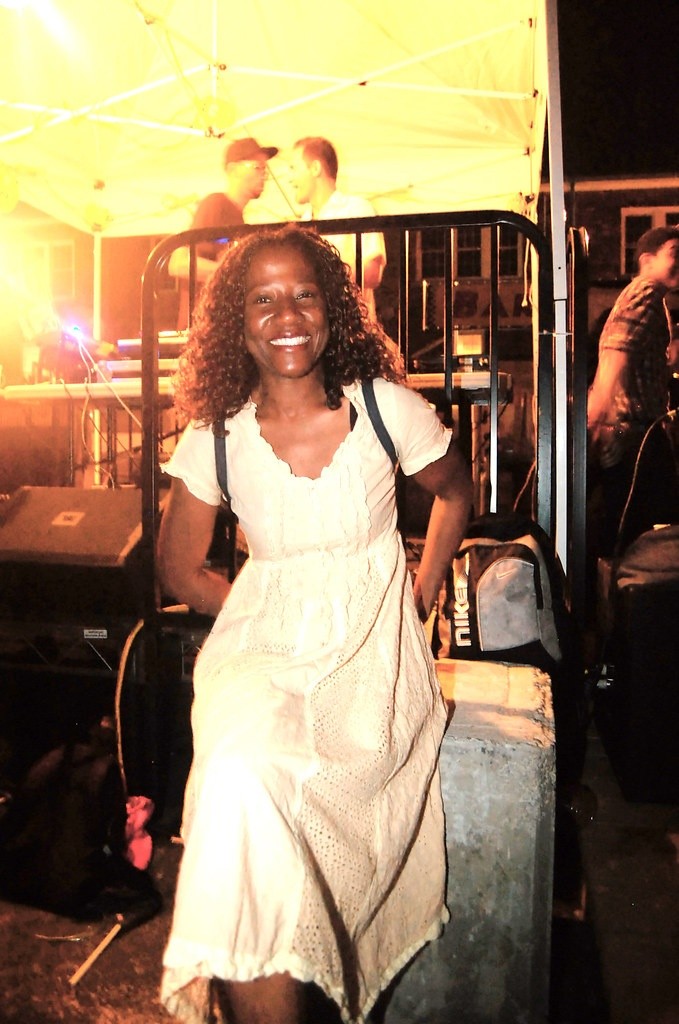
224 138 279 168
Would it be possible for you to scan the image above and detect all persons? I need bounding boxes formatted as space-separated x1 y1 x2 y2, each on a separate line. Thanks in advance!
157 226 473 1024
587 229 679 578
167 137 279 329
291 136 386 323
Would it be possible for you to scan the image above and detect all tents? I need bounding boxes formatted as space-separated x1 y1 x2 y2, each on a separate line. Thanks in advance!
0 0 568 574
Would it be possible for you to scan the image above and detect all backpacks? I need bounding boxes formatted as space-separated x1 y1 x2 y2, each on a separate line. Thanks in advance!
0 741 162 936
440 514 591 735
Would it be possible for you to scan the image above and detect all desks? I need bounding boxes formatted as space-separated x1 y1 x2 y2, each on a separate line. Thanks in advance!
0 371 514 482
